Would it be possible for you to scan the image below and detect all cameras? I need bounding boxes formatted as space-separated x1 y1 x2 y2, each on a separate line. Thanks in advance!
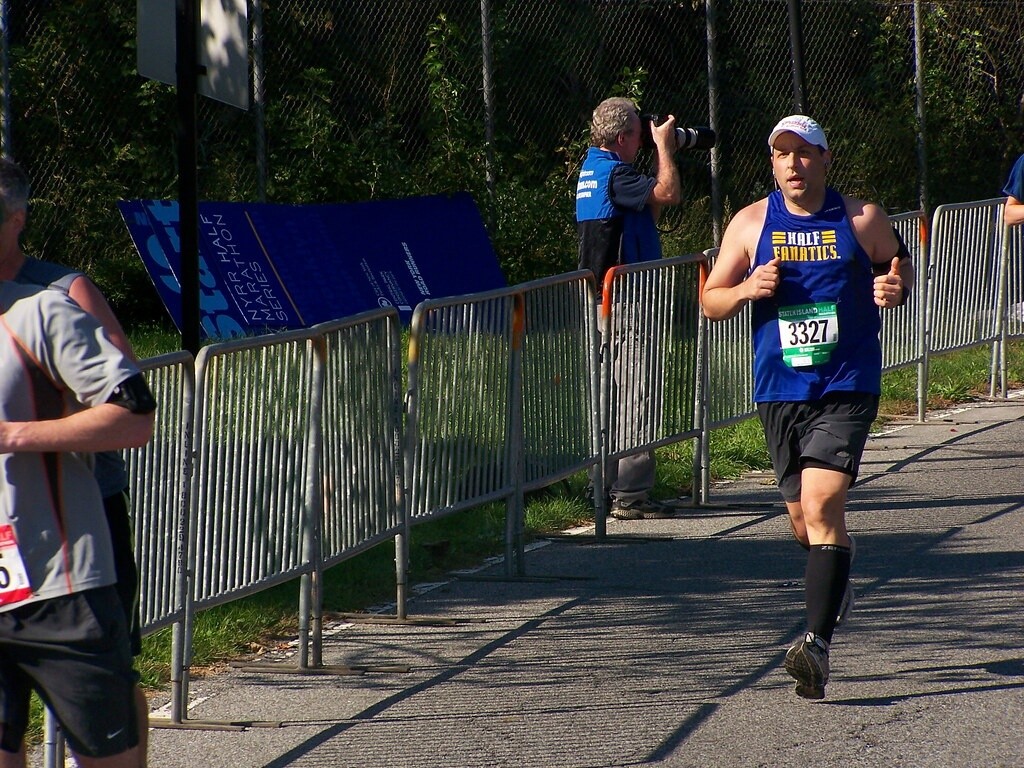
640 113 716 153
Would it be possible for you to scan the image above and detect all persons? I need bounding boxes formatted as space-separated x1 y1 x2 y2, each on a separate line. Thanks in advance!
702 115 913 697
0 154 158 768
1002 154 1024 227
575 97 681 518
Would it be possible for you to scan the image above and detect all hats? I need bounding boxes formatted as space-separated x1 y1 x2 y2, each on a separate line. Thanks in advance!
767 114 828 152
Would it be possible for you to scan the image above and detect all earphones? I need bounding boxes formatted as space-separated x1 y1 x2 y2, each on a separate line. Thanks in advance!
824 160 830 169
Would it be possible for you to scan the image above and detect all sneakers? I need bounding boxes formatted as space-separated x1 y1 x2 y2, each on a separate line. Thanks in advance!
585 489 610 506
785 631 829 699
609 495 675 520
835 534 857 627
